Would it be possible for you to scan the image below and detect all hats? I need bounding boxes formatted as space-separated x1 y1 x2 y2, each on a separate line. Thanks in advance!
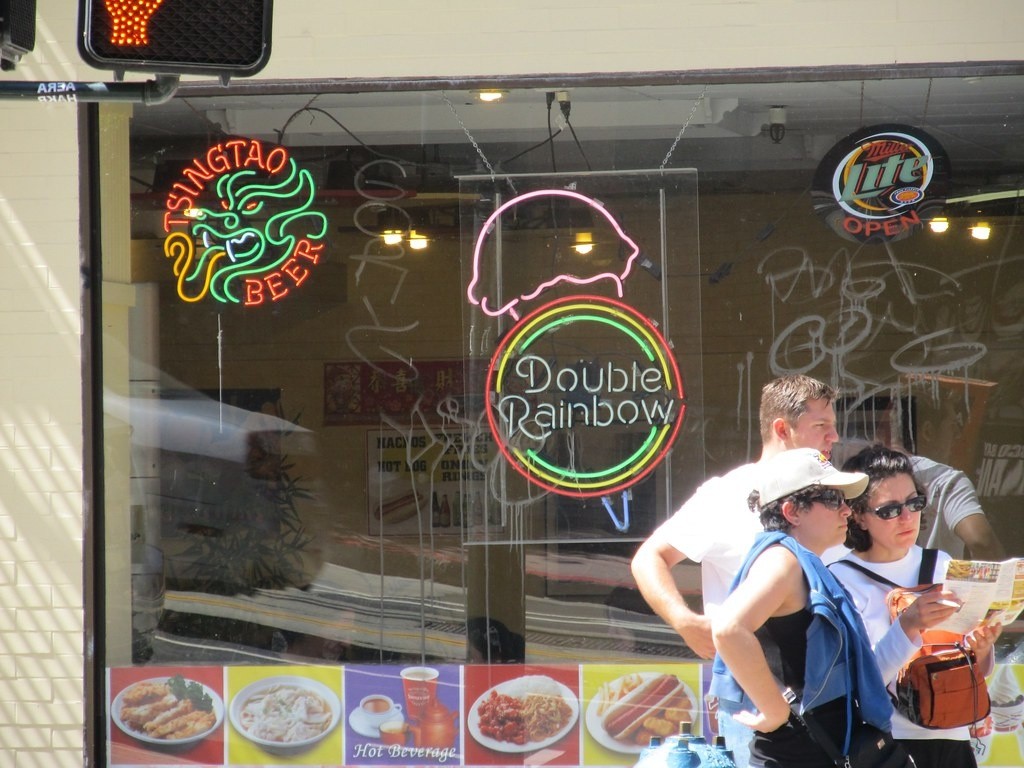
753 447 869 508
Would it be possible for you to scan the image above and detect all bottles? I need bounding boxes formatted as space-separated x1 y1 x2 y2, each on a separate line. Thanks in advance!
462 494 473 528
491 491 501 524
453 492 461 526
472 492 482 525
432 491 440 527
440 495 450 528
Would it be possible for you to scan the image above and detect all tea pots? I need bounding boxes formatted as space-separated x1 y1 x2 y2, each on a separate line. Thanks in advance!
408 698 459 758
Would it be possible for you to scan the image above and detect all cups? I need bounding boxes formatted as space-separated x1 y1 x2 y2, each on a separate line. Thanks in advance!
360 694 402 729
400 666 440 721
377 720 407 748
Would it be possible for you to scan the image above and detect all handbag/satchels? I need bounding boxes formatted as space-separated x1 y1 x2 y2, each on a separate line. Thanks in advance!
835 731 914 767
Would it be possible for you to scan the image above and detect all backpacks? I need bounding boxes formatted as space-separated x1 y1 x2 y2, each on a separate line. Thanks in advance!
822 547 991 728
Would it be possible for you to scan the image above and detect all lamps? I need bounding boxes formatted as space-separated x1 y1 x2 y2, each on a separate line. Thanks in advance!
470 89 509 102
406 225 433 250
928 217 950 234
568 232 600 255
967 220 991 240
378 230 403 245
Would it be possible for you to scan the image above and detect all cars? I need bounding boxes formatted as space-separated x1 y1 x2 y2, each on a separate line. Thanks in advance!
118 349 316 518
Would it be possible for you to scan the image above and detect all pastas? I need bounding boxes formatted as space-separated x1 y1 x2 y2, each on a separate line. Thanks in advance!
514 690 572 744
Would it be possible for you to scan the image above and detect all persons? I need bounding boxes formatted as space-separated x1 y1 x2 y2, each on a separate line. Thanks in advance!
841 388 1007 565
707 447 896 768
824 443 1007 768
631 374 854 768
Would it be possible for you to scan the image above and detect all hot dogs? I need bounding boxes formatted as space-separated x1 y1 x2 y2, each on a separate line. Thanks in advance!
601 672 685 741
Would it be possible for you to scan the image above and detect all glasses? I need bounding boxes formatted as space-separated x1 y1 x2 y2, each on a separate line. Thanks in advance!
860 491 927 519
805 488 845 510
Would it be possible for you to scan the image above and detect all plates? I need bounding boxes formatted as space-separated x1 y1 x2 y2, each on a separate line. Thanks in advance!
468 677 579 754
586 672 698 755
348 706 405 738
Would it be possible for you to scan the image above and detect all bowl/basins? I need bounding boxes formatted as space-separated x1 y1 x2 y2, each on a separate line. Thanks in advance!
229 676 341 755
111 677 224 750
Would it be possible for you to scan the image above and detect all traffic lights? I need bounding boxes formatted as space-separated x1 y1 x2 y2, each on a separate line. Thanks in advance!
78 0 278 76
